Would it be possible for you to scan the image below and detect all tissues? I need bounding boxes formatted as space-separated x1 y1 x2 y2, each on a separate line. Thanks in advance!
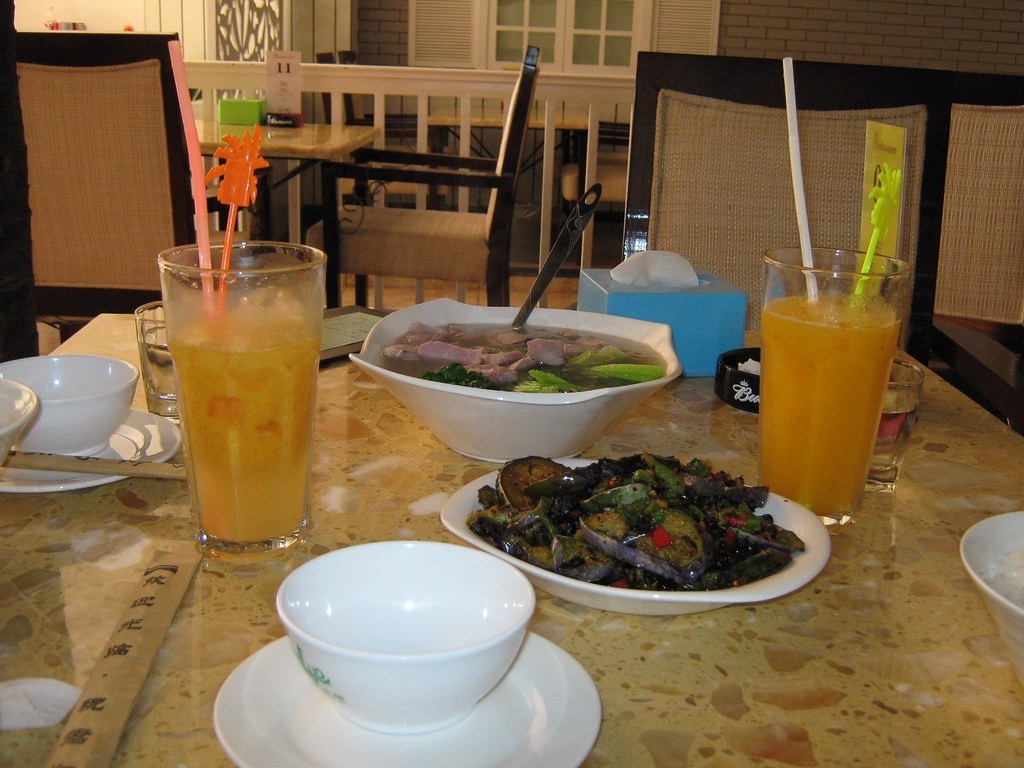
579 248 749 378
218 100 268 127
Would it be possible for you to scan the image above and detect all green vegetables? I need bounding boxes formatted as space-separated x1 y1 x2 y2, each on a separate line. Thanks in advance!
421 346 667 393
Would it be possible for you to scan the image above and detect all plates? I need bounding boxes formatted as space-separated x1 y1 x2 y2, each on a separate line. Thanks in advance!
960 510 1024 618
440 458 830 614
0 409 182 493
213 630 601 768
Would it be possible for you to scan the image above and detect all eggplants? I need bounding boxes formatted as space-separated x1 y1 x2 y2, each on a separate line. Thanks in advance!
489 454 806 584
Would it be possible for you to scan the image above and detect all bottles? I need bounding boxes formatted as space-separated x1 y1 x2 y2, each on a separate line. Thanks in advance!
233 89 244 100
257 90 264 100
45 5 56 32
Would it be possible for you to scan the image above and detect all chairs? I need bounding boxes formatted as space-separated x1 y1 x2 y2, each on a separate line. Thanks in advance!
301 46 539 309
561 125 629 245
314 48 446 207
625 46 1024 438
12 30 200 353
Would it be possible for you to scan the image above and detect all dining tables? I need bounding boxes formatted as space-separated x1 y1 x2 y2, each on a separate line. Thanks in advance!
428 102 591 259
194 118 381 307
0 306 1024 768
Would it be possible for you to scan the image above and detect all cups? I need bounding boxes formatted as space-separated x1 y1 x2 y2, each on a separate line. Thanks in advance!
134 301 180 424
157 241 327 565
865 358 924 493
0 379 39 467
758 246 912 536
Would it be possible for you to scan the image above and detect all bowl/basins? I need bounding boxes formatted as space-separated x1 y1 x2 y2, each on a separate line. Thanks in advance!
0 355 139 457
348 297 682 464
276 541 536 733
